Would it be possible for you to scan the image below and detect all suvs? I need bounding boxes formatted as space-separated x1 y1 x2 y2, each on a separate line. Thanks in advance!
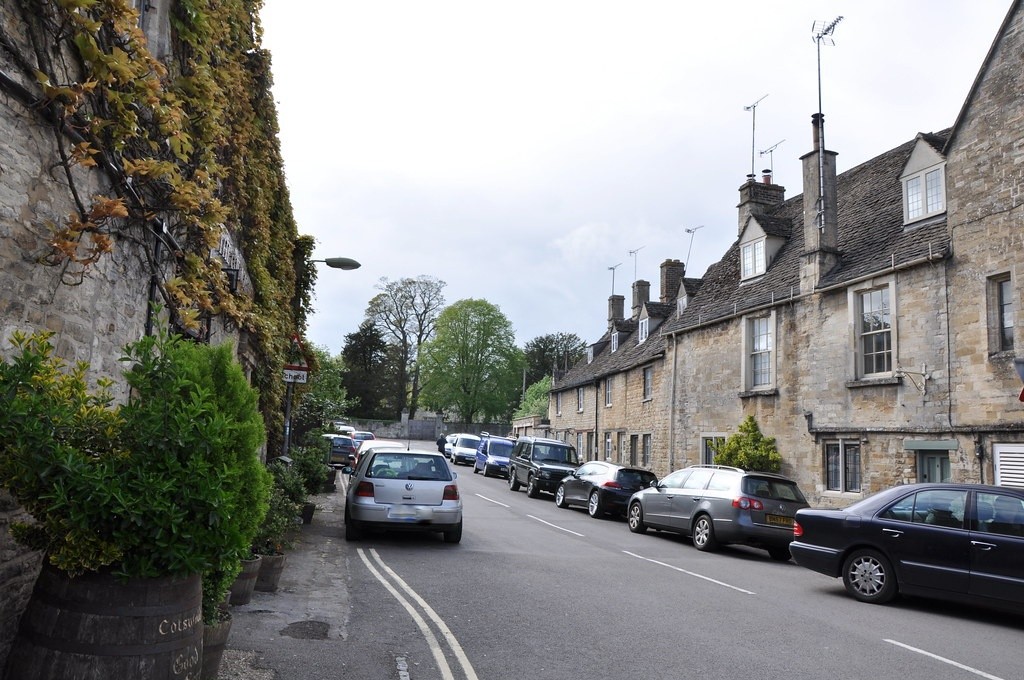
509 436 584 499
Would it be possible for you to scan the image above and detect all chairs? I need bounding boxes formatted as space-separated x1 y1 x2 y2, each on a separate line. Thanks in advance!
535 448 541 458
373 465 390 476
416 463 432 477
549 448 561 460
977 501 995 534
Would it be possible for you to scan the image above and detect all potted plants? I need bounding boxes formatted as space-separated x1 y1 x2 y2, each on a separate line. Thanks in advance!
0 303 331 680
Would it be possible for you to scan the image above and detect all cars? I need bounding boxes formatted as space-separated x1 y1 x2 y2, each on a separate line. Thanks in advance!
554 460 659 519
341 447 463 543
444 434 457 458
627 464 810 563
348 439 409 480
331 421 376 458
788 481 1024 613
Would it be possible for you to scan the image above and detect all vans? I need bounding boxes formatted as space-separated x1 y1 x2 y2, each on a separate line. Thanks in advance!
320 433 352 465
473 431 519 479
450 433 481 465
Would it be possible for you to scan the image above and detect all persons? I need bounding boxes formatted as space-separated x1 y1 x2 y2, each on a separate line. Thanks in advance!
436 433 448 456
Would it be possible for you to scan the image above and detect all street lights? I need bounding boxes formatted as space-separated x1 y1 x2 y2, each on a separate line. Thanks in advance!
283 258 361 458
